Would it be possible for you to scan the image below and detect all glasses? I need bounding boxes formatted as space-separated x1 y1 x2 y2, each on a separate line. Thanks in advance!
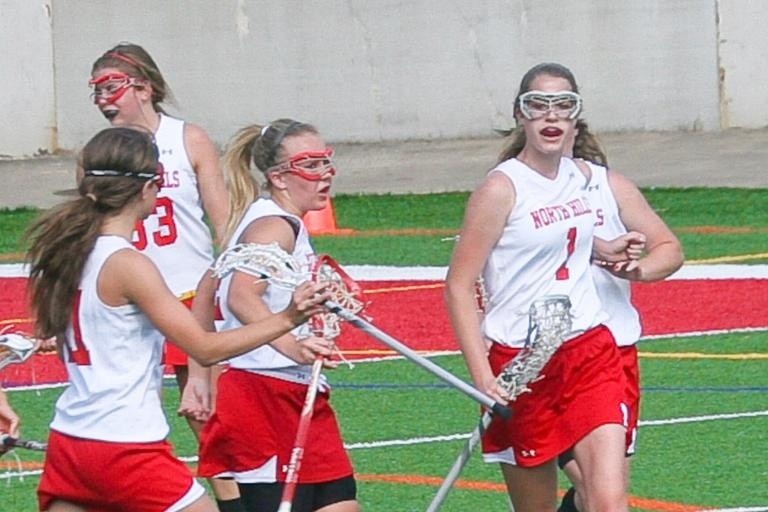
82 162 167 191
87 72 147 106
264 148 339 182
517 89 586 121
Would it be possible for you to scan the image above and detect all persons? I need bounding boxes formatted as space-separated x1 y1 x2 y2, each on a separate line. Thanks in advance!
17 123 337 512
506 95 685 511
171 114 367 512
443 59 650 512
87 37 246 511
0 382 23 442
